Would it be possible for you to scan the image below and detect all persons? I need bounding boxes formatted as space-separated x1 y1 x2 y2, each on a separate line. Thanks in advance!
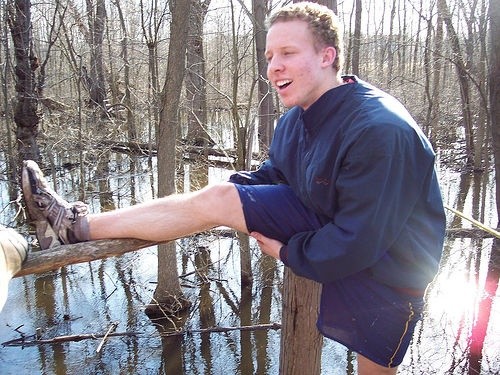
20 0 448 375
0 223 29 314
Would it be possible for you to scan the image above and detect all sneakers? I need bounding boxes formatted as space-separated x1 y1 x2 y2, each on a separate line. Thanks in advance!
20 159 91 251
